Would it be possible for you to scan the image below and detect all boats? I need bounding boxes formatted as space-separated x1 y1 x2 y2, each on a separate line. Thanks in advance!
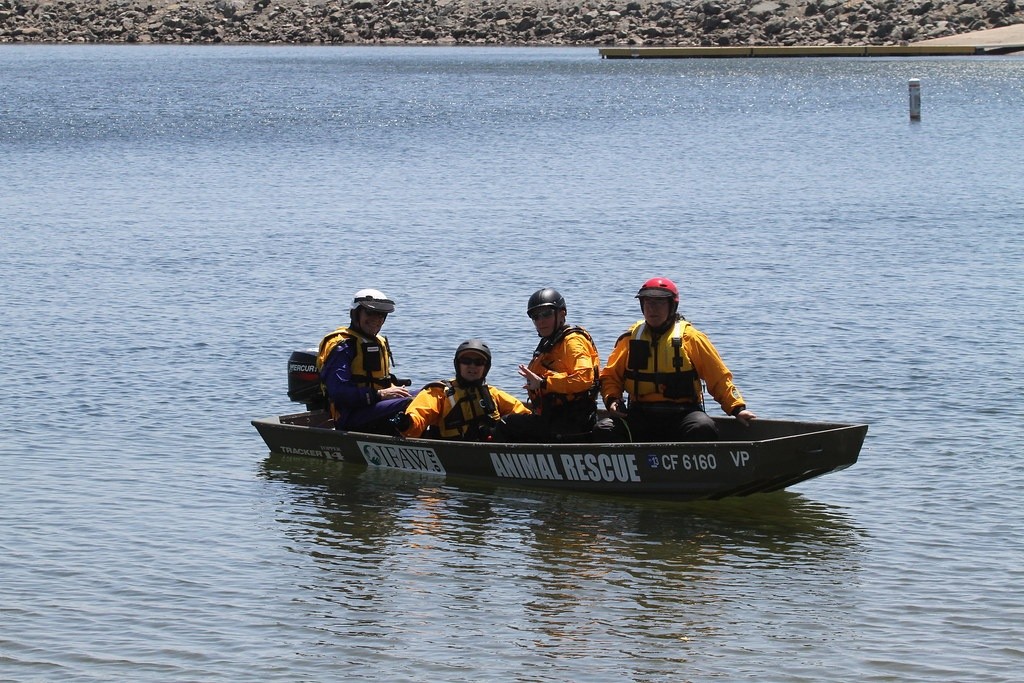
251 408 870 499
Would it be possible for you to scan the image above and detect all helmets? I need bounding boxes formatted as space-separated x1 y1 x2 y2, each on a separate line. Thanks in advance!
349 288 396 318
455 339 491 369
634 277 680 304
526 288 567 316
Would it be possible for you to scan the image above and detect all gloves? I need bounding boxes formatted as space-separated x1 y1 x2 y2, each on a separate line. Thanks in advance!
389 411 411 432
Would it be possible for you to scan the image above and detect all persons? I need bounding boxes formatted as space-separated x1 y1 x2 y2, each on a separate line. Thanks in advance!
517 277 756 442
318 289 421 435
393 338 533 443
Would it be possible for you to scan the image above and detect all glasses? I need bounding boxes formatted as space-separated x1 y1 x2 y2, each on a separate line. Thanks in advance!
459 357 486 366
529 307 557 323
362 307 387 318
643 298 671 308
